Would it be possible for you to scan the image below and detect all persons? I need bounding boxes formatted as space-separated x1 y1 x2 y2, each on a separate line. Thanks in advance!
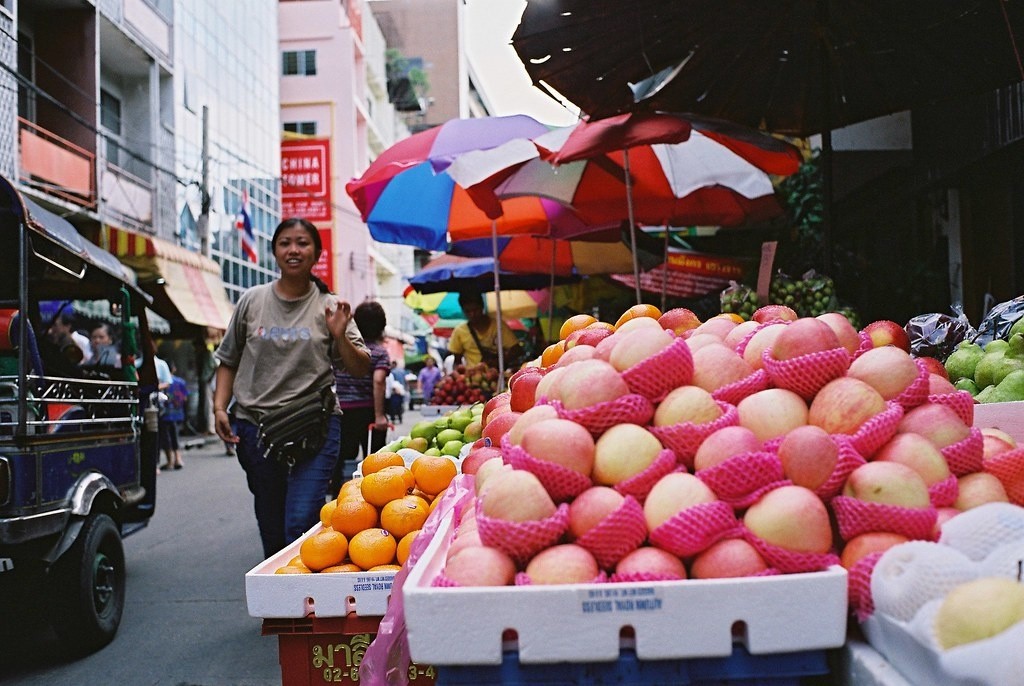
29 300 122 370
190 338 216 435
417 357 441 405
385 362 405 424
213 219 372 562
447 292 519 372
212 371 237 455
332 301 389 502
135 338 183 469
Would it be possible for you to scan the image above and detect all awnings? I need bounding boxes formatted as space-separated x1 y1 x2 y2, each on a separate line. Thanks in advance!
101 223 233 330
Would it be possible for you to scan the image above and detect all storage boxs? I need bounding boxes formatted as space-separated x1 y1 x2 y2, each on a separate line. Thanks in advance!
245 502 1024 686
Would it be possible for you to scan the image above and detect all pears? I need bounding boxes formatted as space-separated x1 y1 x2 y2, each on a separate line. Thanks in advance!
935 556 1024 651
945 312 1024 403
384 400 485 457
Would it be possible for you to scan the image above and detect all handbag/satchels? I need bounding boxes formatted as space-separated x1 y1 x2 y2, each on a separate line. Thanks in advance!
467 322 518 381
236 385 336 474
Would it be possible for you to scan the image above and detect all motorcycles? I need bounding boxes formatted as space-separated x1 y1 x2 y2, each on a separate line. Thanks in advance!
0 172 161 657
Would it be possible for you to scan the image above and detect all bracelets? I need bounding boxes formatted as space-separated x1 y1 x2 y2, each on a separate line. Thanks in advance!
213 407 226 413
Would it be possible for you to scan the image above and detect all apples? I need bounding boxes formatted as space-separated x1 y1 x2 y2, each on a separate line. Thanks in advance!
953 427 1024 510
441 317 981 607
750 305 798 324
428 361 497 405
657 307 702 336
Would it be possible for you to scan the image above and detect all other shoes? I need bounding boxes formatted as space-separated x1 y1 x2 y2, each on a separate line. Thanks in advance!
174 463 182 469
227 449 236 456
160 465 174 471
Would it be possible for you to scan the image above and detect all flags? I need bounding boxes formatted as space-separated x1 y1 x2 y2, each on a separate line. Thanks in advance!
237 192 257 263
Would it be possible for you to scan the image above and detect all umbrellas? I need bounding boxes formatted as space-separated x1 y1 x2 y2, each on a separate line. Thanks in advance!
345 0 1024 392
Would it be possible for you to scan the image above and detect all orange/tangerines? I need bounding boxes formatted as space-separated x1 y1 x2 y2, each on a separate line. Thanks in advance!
270 453 456 573
541 304 661 365
719 276 860 330
